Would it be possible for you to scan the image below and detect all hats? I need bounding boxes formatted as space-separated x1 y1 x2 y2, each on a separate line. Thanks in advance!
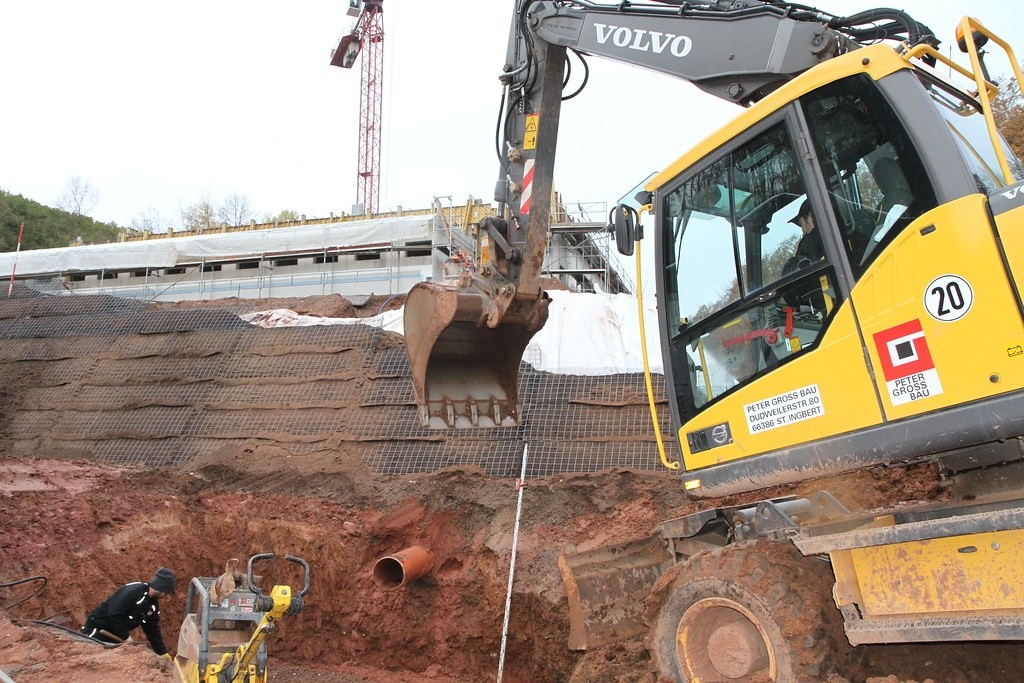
787 193 836 227
149 567 176 592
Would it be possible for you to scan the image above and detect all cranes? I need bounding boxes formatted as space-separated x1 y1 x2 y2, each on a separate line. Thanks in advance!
330 0 383 215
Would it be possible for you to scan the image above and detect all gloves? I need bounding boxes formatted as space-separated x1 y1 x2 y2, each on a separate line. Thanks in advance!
161 653 172 661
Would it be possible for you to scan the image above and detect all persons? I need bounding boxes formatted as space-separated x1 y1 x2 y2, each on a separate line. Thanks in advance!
761 192 870 369
78 566 177 661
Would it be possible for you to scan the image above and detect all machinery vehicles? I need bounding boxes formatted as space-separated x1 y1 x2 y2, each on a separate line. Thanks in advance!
400 0 1024 683
